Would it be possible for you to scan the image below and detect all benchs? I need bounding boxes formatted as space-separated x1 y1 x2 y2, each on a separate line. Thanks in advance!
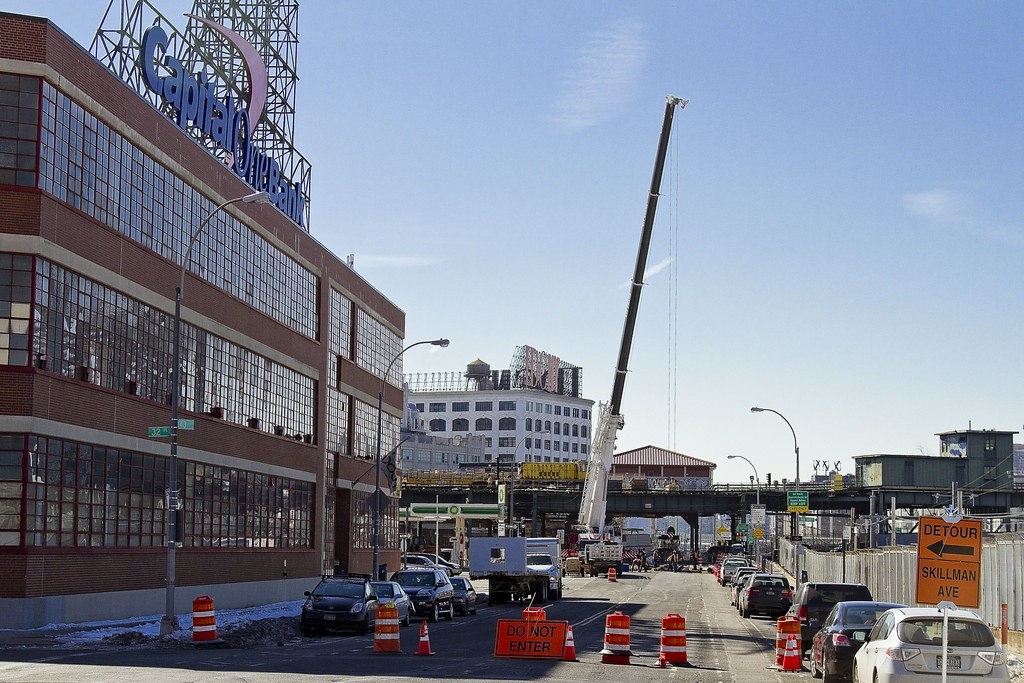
755 581 782 586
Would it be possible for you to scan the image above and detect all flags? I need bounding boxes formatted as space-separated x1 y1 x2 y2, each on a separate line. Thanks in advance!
381 448 396 493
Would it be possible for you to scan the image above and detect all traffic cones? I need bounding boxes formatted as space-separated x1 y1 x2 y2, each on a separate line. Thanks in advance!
559 626 580 662
791 633 804 669
777 635 802 673
413 620 436 656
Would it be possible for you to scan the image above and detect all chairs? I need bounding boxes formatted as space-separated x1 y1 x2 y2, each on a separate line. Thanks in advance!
848 615 862 624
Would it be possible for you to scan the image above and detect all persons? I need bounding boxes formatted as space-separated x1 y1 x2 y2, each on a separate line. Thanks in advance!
667 551 679 572
631 548 659 572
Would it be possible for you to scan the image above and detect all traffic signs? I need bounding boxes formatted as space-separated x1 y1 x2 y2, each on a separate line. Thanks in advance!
915 514 983 609
399 533 413 539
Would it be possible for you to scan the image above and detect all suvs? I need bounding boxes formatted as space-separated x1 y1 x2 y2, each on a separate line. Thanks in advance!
388 566 455 623
780 582 877 661
400 552 463 577
738 572 795 620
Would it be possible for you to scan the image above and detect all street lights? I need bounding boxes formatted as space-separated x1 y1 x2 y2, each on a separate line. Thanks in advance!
750 407 800 543
509 430 552 537
727 455 760 565
158 189 272 617
373 337 450 580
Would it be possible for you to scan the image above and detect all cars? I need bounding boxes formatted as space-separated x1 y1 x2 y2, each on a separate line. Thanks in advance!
369 581 414 628
447 576 478 617
809 601 928 683
852 607 1012 683
300 574 380 637
705 543 775 610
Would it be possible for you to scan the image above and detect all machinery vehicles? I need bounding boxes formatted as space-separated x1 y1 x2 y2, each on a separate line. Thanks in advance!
554 92 692 578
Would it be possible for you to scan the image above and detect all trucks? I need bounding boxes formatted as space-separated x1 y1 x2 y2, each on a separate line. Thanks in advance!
513 537 566 604
